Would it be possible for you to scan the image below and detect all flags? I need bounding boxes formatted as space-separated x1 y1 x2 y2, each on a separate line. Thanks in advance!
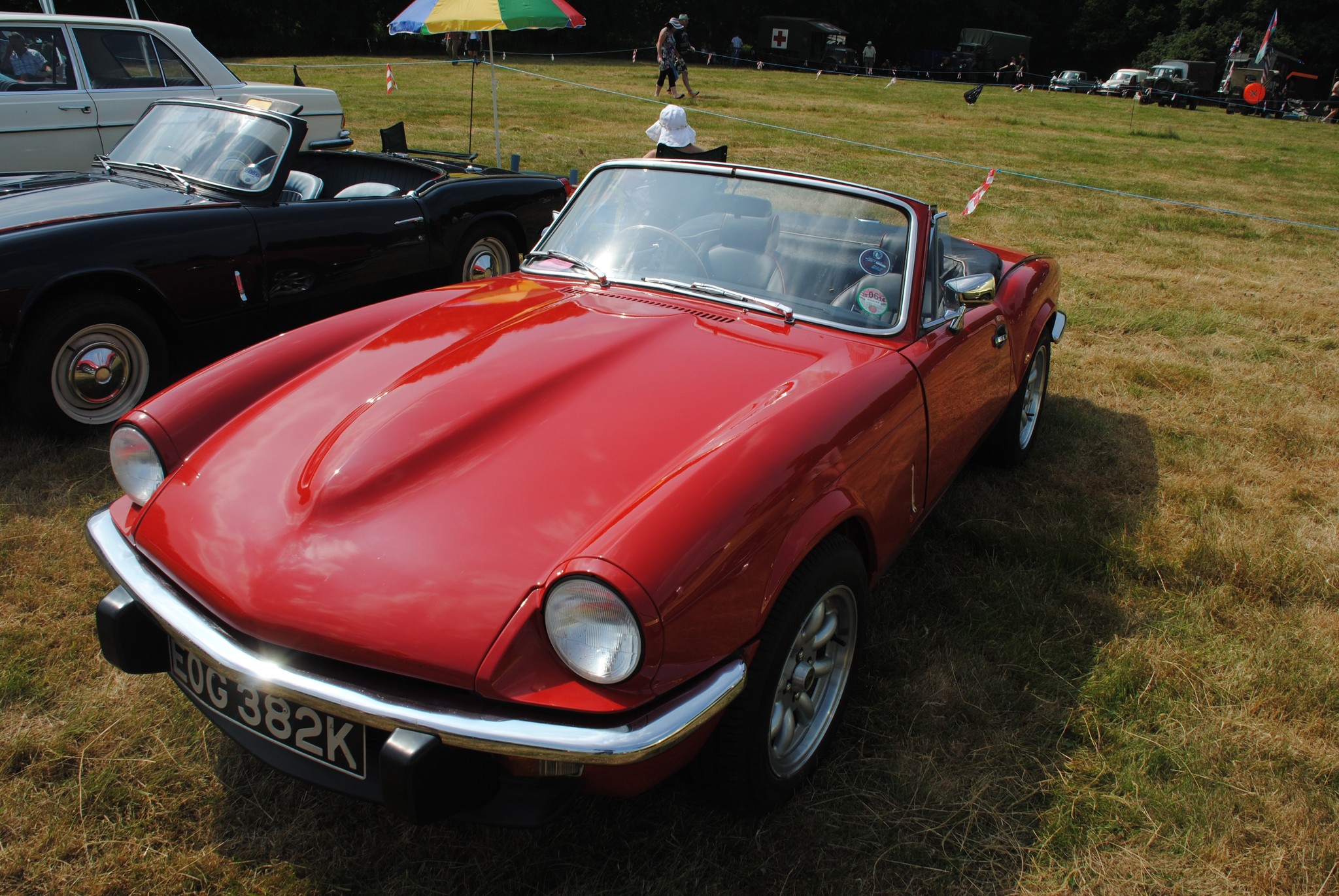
1222 8 1277 92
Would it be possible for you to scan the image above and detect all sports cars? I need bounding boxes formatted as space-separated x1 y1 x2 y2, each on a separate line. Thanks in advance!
0 97 573 439
83 156 1068 820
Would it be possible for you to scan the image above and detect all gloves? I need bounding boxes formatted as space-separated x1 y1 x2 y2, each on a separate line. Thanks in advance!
690 46 695 53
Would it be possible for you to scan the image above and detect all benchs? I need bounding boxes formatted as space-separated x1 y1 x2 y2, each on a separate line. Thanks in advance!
647 208 1003 308
92 75 199 89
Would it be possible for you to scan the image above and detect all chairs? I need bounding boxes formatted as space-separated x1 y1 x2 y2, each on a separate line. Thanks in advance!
829 233 945 326
0 79 25 91
252 170 324 202
697 210 786 296
333 182 400 198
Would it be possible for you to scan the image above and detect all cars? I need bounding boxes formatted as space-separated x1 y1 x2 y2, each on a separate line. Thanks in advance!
1099 68 1149 98
0 11 355 173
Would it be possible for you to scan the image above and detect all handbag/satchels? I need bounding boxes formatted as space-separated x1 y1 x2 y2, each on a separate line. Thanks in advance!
964 83 984 104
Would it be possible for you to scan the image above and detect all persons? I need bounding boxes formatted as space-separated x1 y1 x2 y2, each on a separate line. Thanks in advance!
863 41 876 75
444 32 463 66
730 34 743 67
465 31 483 66
654 14 700 99
1321 81 1339 124
999 52 1029 85
1 32 54 79
641 105 703 161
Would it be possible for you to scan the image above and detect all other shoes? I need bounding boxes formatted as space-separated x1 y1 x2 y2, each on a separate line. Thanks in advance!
667 90 671 93
674 94 685 99
456 58 461 64
689 91 699 98
1322 119 1327 124
452 61 457 66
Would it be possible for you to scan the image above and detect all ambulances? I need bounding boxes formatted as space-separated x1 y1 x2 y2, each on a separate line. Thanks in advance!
749 15 863 75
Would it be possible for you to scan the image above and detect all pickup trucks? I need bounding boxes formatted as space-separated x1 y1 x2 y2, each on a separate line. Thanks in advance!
1050 71 1097 92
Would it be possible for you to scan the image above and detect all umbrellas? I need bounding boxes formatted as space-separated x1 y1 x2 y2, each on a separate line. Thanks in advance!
387 0 587 169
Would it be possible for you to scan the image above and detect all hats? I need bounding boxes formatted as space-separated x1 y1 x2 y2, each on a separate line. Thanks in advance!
886 59 889 63
668 17 684 29
678 14 689 20
1051 71 1056 73
866 41 872 44
646 105 696 147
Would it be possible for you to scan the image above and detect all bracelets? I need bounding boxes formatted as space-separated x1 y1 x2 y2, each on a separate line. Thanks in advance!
459 38 461 39
480 40 481 41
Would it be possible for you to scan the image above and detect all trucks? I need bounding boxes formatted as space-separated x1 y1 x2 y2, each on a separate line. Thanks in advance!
1220 46 1307 117
950 28 1033 84
1136 58 1218 110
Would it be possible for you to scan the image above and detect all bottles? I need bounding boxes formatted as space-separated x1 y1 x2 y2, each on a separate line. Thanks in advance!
459 38 461 45
465 44 467 50
